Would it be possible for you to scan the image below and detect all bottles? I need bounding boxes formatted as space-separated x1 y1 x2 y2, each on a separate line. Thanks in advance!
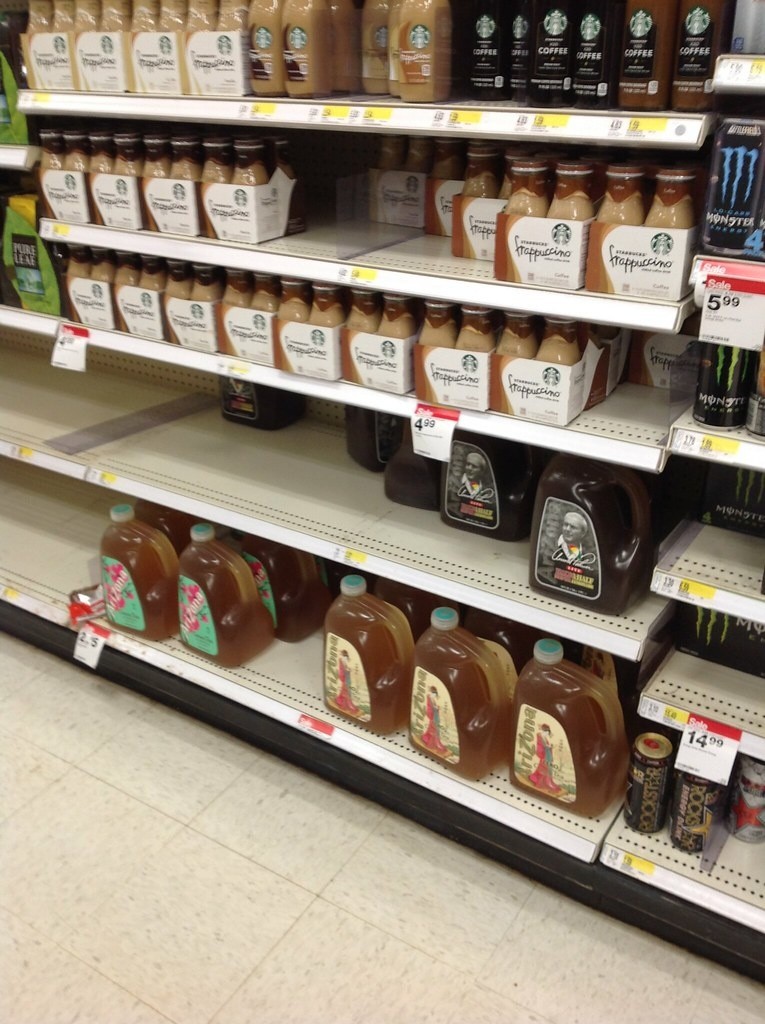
26 0 728 110
36 126 299 186
217 374 301 430
373 132 696 231
66 242 583 366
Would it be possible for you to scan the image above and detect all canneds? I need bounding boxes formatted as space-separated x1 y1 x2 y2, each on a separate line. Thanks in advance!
625 735 765 853
691 119 765 442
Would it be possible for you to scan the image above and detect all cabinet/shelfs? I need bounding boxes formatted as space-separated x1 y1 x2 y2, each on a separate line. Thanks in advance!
0 143 217 638
15 91 721 896
590 51 765 986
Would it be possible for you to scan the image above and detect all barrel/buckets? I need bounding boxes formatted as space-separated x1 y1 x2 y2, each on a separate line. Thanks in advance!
529 447 653 614
442 427 541 541
344 404 447 513
101 498 329 668
324 571 630 816
